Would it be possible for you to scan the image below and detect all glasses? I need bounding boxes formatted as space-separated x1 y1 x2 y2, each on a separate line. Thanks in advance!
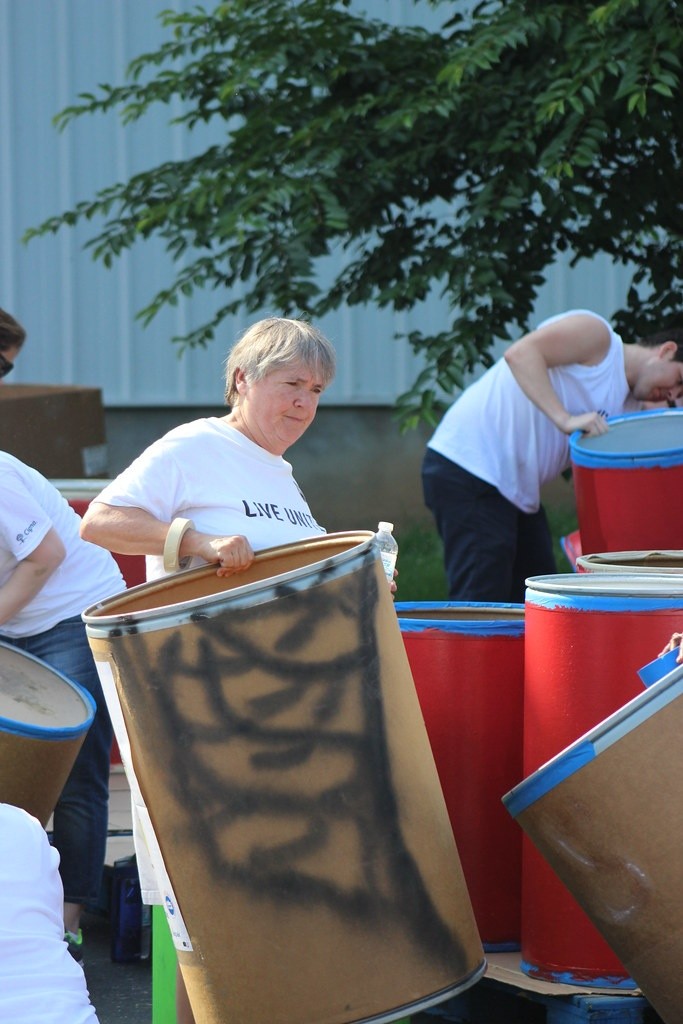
0 354 14 379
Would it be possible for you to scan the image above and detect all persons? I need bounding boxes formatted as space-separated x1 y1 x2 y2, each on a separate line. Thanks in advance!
78 315 402 1023
0 801 103 1024
0 450 130 967
419 308 683 604
0 308 27 385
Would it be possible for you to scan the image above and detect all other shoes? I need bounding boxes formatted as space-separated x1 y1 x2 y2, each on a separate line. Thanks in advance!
64 929 85 968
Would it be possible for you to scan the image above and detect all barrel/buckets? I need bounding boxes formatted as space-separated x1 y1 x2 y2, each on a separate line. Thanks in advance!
80 532 489 1024
522 573 683 987
393 602 525 957
571 406 683 549
501 664 683 1024
577 550 683 572
0 642 96 826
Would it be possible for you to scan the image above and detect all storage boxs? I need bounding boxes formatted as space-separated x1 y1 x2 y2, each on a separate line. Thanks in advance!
0 382 110 477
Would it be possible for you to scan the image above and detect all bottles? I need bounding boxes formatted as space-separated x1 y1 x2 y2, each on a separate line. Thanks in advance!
374 521 398 582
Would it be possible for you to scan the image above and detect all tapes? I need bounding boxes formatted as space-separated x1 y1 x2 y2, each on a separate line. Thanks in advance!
162 517 196 573
